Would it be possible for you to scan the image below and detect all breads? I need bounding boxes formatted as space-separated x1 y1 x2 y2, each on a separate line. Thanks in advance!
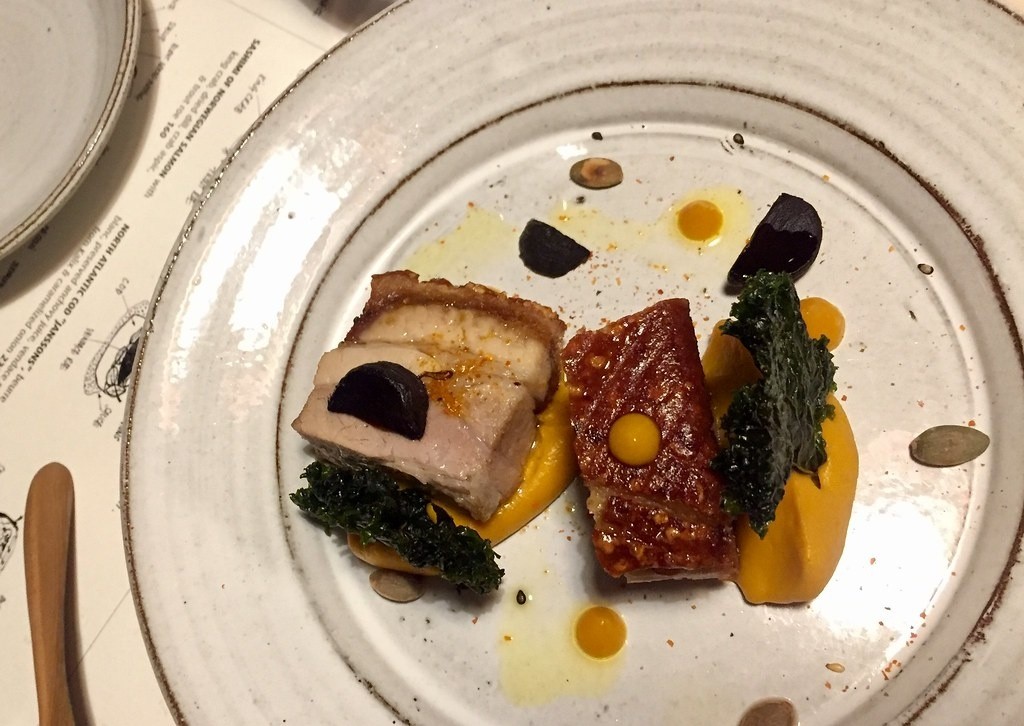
564 298 738 582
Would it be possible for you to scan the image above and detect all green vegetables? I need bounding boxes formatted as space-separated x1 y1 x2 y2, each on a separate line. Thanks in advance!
289 460 504 591
713 271 840 537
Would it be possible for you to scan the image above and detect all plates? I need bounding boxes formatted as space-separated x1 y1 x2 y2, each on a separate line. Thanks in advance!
122 1 1024 726
0 1 143 256
